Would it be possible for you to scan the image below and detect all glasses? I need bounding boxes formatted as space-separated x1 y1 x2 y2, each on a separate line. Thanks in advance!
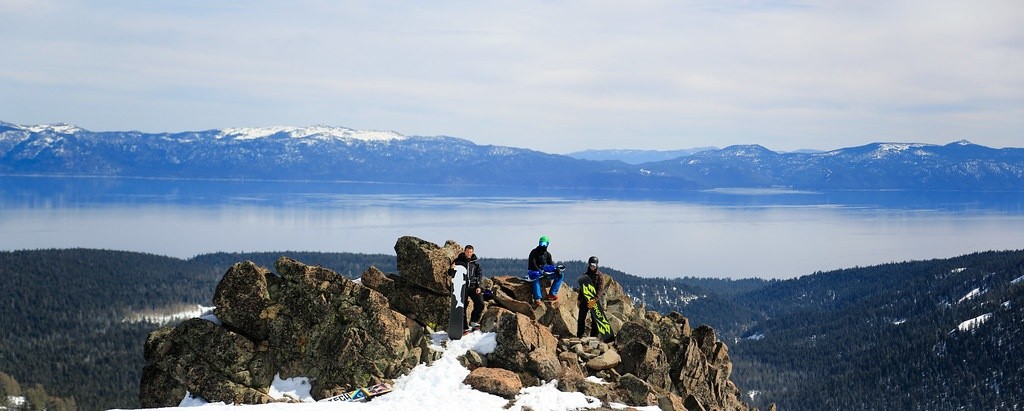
589 263 596 266
539 241 548 247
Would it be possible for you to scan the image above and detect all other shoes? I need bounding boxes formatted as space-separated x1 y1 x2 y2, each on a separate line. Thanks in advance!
463 329 469 335
469 321 481 328
534 299 541 305
547 295 558 300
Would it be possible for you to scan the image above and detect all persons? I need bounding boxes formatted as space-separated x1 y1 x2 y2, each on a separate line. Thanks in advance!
527 236 564 305
577 256 604 338
448 245 485 335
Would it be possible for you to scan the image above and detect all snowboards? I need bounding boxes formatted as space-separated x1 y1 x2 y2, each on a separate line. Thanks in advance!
519 263 565 282
448 261 467 340
579 275 615 342
315 381 394 403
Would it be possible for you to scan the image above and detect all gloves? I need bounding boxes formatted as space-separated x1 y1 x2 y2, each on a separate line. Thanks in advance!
587 299 596 308
577 301 581 307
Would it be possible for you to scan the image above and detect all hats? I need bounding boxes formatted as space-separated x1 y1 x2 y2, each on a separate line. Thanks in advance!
588 256 599 264
540 236 549 243
